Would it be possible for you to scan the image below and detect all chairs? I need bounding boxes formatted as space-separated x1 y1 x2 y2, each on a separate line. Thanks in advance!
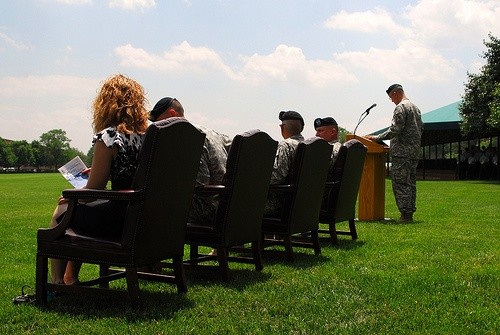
36 128 368 313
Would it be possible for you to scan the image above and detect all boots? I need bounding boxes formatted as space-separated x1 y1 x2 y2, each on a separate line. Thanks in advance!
397 213 413 222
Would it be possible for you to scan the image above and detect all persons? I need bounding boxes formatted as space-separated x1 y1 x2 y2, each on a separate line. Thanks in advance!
263 110 304 215
148 97 225 227
367 84 422 223
456 143 498 165
49 75 155 285
314 117 341 162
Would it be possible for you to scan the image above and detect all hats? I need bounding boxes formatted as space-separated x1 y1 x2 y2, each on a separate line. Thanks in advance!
386 84 402 95
279 111 304 125
148 97 175 122
314 117 337 129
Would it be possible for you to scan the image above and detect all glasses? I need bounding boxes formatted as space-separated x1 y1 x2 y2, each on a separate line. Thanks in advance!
389 94 394 100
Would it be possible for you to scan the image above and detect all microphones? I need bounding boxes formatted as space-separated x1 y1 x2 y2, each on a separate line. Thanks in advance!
363 103 376 115
